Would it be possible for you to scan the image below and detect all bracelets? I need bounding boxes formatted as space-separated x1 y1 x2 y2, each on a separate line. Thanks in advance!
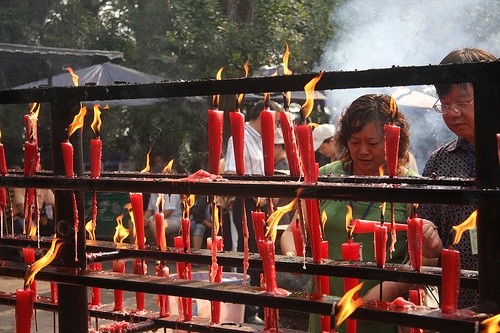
396 282 401 297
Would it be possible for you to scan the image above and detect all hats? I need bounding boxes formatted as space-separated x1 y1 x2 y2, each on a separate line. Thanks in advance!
274 128 284 145
313 124 335 151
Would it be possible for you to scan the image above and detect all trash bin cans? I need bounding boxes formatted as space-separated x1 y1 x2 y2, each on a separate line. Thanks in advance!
168 272 250 333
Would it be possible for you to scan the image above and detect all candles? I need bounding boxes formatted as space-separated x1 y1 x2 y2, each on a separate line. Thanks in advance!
0 43 460 333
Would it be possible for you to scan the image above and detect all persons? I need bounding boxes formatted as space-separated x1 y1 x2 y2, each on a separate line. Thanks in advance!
129 156 225 268
280 95 443 333
412 48 498 310
6 162 54 237
225 99 419 325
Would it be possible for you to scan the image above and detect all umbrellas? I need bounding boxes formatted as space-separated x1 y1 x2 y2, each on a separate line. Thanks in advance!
0 62 442 109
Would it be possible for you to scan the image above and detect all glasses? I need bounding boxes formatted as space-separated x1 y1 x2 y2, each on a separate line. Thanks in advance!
432 98 474 114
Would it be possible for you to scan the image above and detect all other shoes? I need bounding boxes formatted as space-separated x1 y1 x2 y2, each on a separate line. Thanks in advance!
246 315 264 325
